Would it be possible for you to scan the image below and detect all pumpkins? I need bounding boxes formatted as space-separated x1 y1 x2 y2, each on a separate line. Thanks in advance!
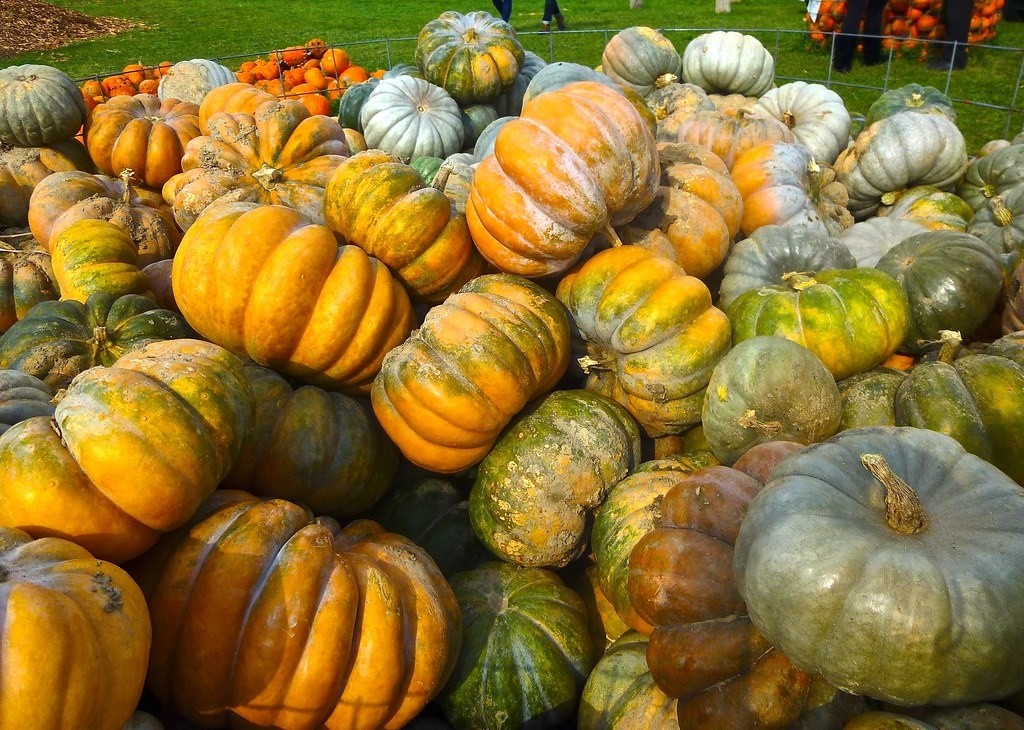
0 0 1024 730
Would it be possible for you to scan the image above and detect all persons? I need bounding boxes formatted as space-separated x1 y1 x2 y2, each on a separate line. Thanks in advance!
492 0 512 23
833 0 894 73
541 0 566 32
930 0 975 69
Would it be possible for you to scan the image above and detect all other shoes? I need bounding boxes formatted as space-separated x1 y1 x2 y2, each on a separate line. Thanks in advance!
556 16 564 30
536 23 550 35
928 63 960 70
865 54 894 66
833 59 852 73
